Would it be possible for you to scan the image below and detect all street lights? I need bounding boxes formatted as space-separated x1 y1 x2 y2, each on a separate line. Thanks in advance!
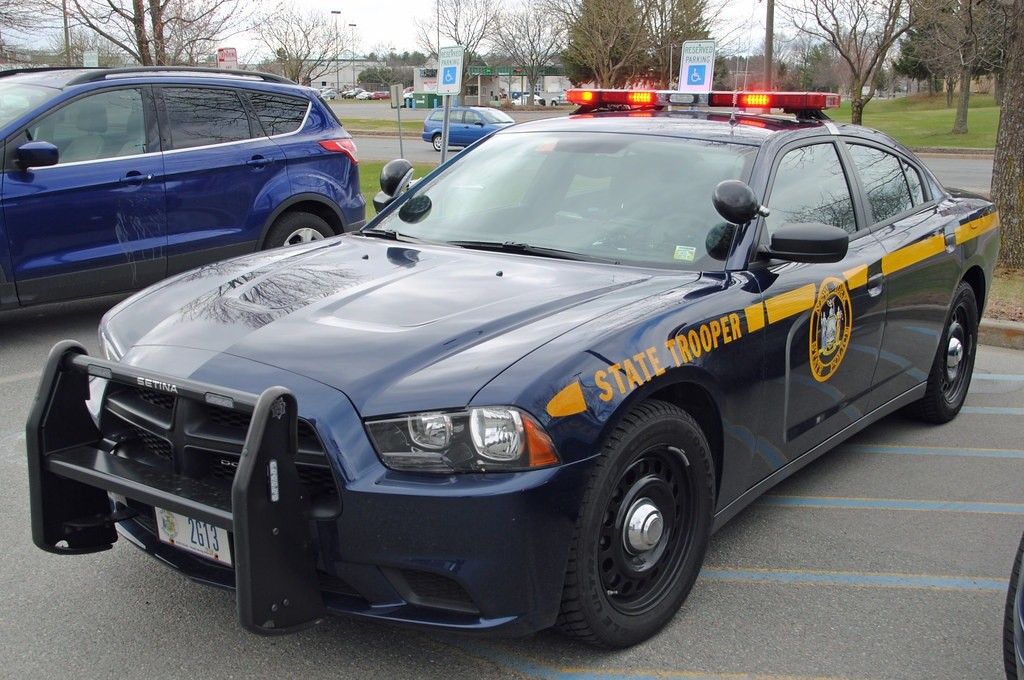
331 10 341 90
348 23 357 91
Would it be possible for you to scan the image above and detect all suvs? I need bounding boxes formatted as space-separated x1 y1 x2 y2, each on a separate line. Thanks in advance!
0 64 371 326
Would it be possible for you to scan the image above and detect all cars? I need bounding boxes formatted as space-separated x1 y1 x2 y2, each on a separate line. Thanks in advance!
510 93 545 106
549 92 574 106
318 87 414 100
421 106 519 152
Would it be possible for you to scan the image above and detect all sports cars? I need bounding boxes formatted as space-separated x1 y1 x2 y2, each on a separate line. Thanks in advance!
22 86 1004 654
